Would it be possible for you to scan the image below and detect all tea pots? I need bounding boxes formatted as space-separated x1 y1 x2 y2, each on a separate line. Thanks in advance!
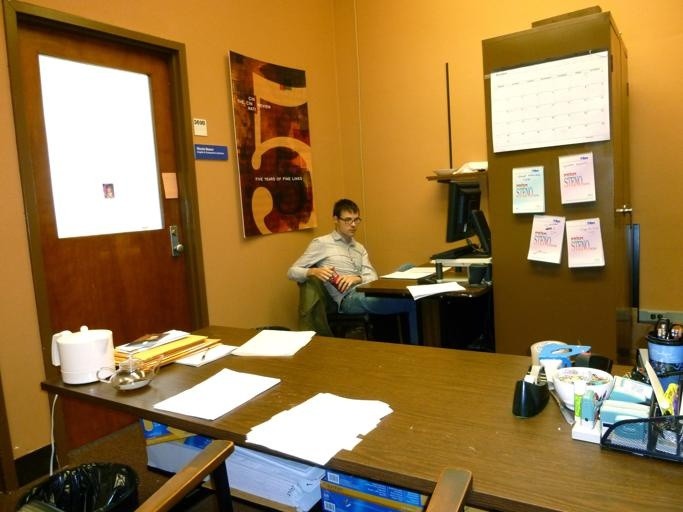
96 352 166 391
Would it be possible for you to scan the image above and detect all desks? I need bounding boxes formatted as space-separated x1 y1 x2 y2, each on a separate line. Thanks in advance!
40 325 683 512
357 262 491 349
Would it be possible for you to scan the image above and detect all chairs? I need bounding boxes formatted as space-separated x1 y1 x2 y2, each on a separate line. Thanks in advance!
303 263 404 343
133 438 473 512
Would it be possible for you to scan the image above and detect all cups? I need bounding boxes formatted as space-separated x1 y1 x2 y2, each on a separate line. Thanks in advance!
530 341 568 368
642 333 683 424
328 273 347 294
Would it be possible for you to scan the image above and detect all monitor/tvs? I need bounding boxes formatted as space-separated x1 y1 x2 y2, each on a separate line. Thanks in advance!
446 182 491 259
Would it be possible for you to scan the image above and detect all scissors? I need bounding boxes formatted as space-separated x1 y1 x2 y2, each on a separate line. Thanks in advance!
653 318 683 339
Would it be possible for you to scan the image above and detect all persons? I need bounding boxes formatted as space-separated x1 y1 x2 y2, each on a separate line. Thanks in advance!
286 198 419 346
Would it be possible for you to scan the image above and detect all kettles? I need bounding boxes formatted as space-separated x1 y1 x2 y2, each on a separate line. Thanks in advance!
49 325 116 386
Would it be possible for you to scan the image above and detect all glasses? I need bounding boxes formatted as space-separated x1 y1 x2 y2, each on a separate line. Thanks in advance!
339 216 361 225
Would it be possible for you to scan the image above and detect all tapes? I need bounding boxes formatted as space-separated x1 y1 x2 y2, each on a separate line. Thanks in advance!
524 366 541 384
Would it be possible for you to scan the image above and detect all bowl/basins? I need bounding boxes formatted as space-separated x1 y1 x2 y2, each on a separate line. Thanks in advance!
553 367 612 411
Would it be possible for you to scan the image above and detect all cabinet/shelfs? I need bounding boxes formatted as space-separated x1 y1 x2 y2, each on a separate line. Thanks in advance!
481 5 636 365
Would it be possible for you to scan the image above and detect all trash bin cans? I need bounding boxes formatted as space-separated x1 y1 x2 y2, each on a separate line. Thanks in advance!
17 462 140 512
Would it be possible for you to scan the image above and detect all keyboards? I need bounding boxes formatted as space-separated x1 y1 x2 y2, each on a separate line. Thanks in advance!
432 246 473 259
